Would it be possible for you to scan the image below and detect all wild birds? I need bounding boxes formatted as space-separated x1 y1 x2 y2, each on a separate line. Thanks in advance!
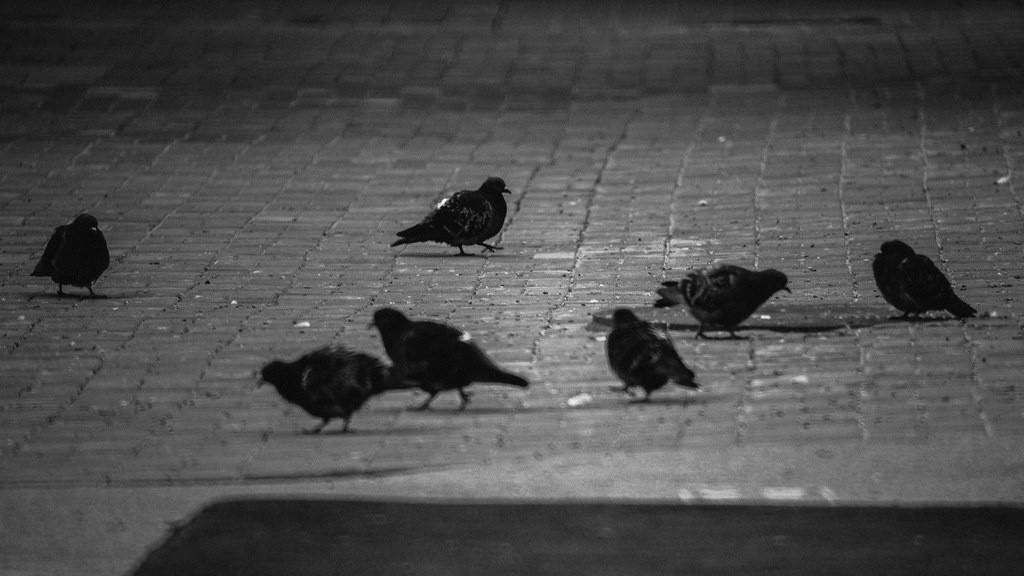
260 308 528 434
32 214 109 297
606 306 701 403
872 240 976 320
389 176 510 255
656 263 792 341
